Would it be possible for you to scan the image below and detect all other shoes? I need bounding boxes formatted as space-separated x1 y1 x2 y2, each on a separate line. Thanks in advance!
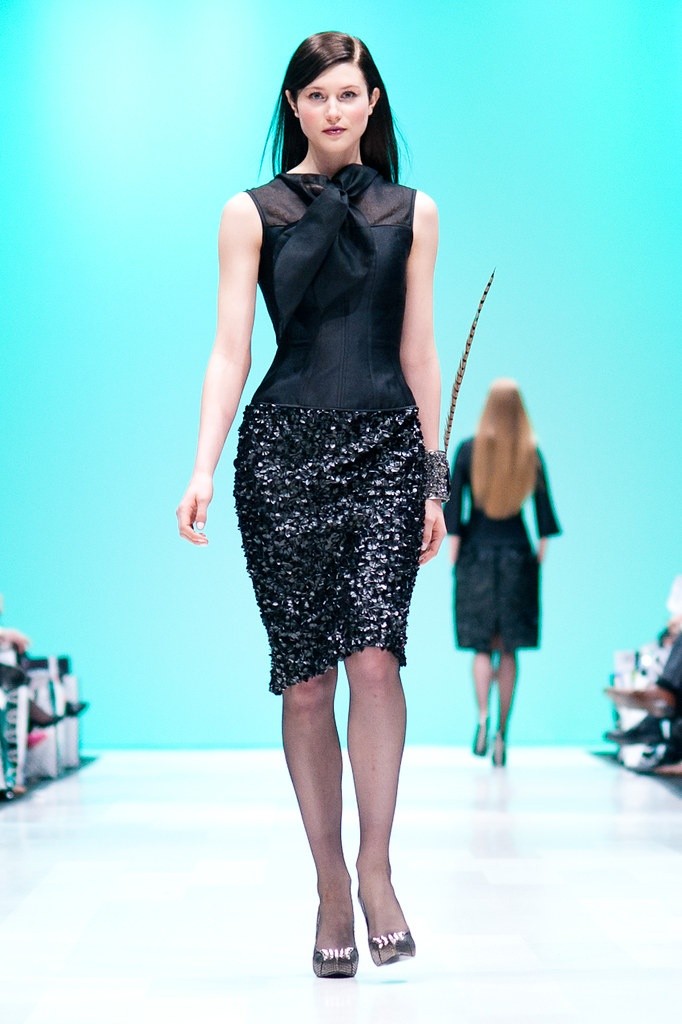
26 733 45 747
606 717 665 745
654 763 682 776
630 756 680 777
0 786 23 801
607 684 672 716
68 700 88 716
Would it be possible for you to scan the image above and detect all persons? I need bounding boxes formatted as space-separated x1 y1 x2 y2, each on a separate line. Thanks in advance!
445 378 561 765
603 619 682 774
0 627 88 800
175 30 450 978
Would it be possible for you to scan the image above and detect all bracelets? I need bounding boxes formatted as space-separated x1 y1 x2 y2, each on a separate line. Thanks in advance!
422 450 449 504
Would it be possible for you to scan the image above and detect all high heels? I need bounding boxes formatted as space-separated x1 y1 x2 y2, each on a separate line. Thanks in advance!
28 701 65 733
357 890 416 966
313 904 359 979
474 716 490 755
492 732 506 765
